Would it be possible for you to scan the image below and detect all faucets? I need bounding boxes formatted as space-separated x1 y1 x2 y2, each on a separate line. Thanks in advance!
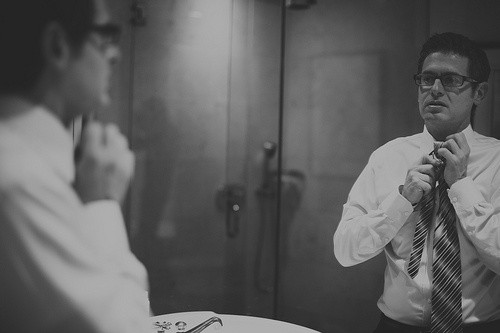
184 316 224 333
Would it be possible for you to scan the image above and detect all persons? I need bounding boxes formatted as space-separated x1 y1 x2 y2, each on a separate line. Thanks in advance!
0 0 154 333
332 33 500 333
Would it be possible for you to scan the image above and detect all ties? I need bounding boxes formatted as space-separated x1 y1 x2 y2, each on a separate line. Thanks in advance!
408 141 464 333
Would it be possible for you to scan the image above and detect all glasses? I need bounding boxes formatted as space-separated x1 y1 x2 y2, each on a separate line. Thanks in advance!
414 73 480 87
66 22 122 40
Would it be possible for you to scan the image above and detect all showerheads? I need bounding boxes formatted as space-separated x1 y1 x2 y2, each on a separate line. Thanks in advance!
260 141 277 178
